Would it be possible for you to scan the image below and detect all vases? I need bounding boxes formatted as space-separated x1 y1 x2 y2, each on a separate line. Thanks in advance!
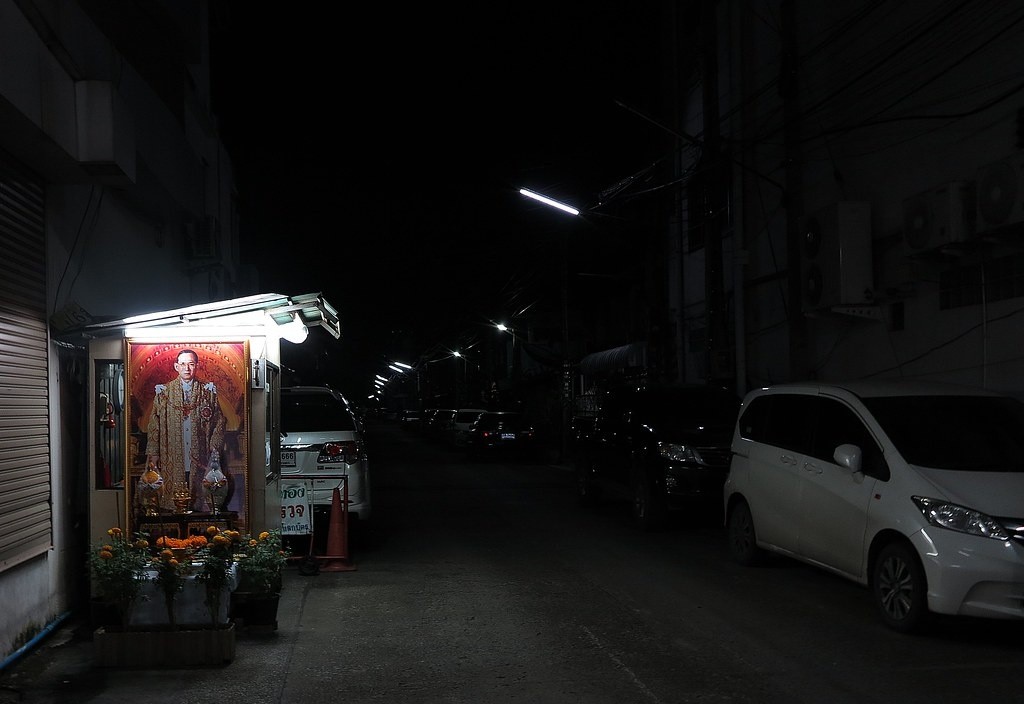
93 566 286 667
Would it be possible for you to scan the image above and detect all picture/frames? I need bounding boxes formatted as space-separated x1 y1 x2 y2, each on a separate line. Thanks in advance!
125 339 250 547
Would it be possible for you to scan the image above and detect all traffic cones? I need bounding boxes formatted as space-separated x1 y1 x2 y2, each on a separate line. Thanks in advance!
315 487 359 571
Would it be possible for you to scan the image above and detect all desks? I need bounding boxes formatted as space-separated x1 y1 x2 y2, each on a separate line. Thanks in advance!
136 512 239 536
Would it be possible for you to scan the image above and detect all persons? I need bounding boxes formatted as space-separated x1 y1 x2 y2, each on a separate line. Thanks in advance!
145 350 226 514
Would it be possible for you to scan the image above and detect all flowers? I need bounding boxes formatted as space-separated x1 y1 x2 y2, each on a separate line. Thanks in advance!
87 524 292 630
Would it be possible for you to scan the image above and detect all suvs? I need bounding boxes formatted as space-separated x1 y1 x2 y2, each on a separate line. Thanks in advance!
266 383 371 532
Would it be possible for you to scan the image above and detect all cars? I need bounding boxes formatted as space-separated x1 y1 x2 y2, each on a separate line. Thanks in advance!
721 382 1024 636
365 381 752 537
